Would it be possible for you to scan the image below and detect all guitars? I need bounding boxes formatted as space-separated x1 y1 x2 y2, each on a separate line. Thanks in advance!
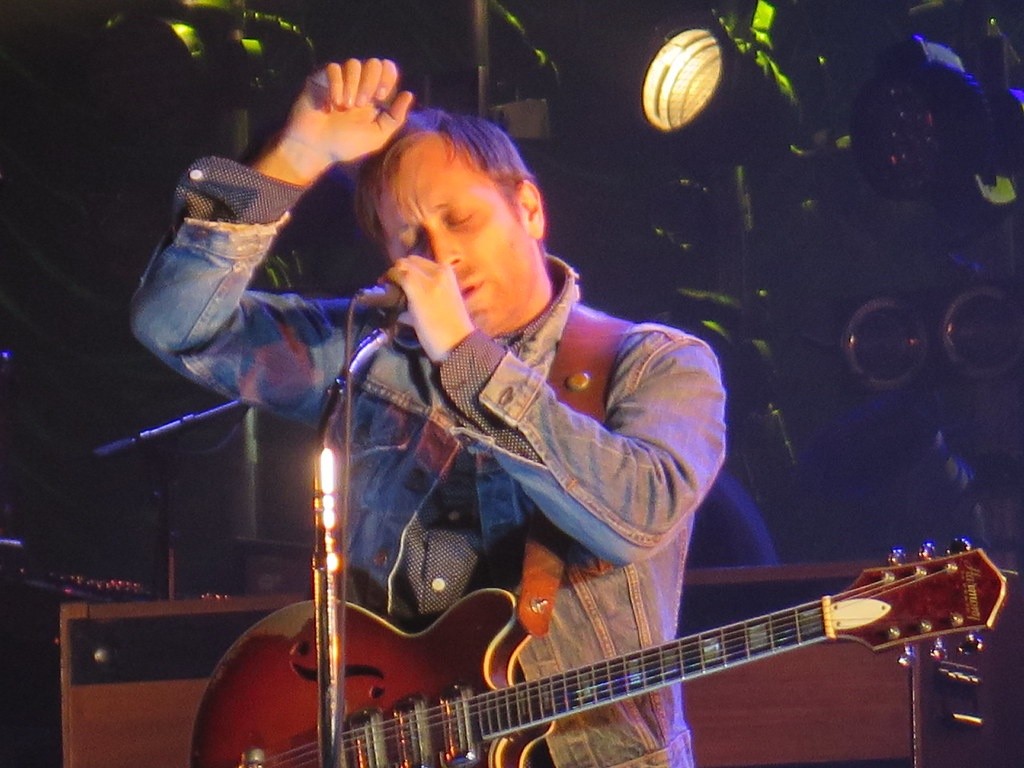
188 536 1011 768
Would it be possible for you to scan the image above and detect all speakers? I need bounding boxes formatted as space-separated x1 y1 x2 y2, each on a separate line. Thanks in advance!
60 592 306 768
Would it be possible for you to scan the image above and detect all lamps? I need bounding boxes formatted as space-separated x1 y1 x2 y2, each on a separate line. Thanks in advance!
636 18 804 163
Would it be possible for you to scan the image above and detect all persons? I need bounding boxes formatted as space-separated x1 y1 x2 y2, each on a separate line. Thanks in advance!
126 58 727 768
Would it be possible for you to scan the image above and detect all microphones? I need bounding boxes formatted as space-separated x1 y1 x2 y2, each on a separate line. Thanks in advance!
354 281 410 312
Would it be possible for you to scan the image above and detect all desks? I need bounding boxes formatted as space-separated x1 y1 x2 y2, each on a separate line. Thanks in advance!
0 556 923 768
